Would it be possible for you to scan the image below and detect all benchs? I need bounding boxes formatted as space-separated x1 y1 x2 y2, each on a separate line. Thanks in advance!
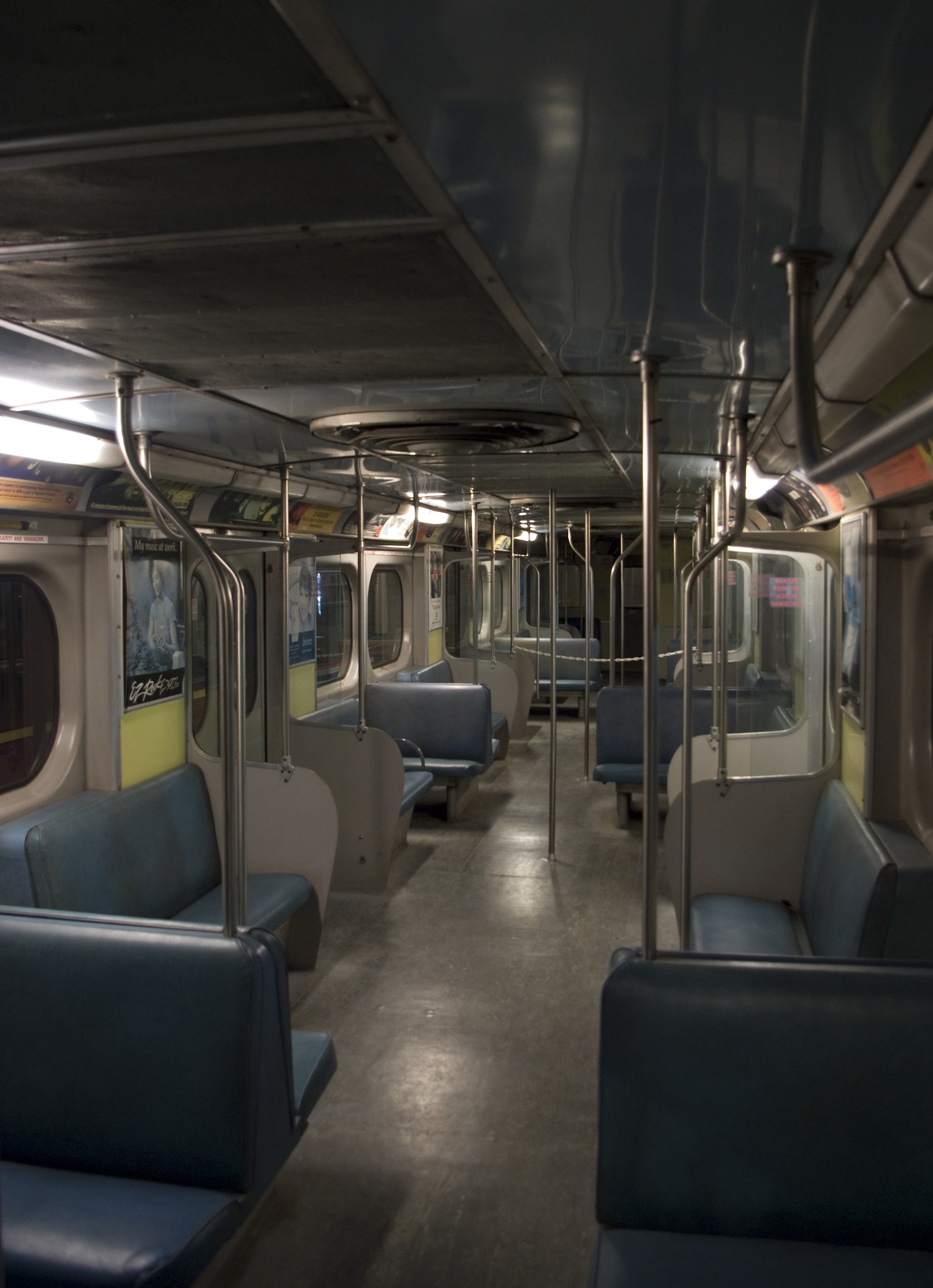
0 634 933 1288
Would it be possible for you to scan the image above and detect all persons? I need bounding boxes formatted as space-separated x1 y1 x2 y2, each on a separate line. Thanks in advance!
149 560 180 665
292 564 314 632
